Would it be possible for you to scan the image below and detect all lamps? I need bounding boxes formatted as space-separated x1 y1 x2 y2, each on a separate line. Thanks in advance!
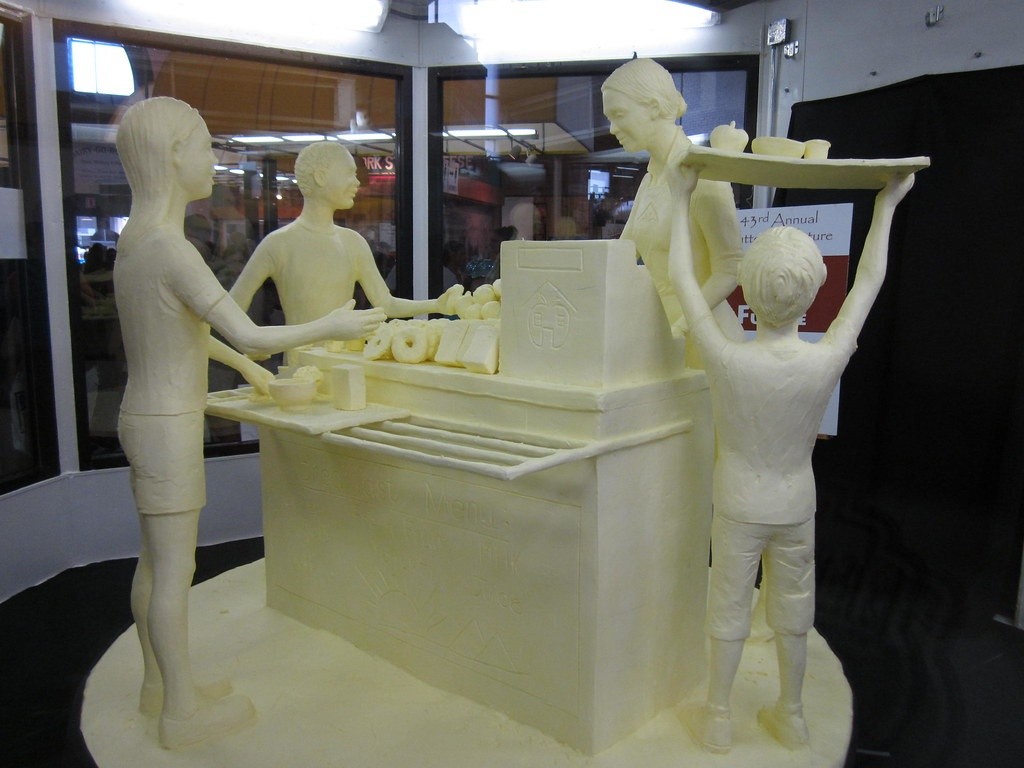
526 147 536 166
510 136 521 160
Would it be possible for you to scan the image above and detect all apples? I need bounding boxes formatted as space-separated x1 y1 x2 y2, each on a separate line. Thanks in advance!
709 119 749 153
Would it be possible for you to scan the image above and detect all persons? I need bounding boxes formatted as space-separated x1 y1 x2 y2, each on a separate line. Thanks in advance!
116 97 388 750
600 59 781 674
666 140 914 754
442 226 520 295
0 215 397 476
231 140 473 360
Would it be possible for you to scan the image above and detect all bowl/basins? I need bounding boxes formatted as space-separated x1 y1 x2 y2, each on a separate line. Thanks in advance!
270 377 316 412
752 137 804 159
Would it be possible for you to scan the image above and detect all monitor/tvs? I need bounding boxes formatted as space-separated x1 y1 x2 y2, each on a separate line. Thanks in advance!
66 37 135 96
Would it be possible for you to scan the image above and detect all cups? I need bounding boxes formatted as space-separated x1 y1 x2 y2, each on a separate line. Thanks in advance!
804 140 831 160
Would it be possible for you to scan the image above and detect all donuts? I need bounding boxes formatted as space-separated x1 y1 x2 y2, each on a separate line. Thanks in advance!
362 319 449 363
